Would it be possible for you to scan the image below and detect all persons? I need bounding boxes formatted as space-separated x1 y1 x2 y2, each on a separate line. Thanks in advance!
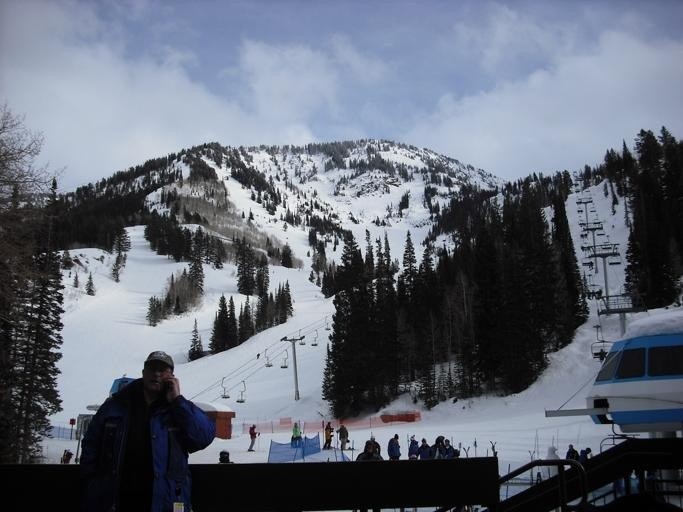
218 451 229 464
600 349 604 363
81 351 216 512
322 422 591 512
248 424 257 451
293 423 301 447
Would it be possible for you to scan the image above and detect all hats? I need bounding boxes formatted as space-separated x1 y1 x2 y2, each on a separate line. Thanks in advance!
144 350 174 369
